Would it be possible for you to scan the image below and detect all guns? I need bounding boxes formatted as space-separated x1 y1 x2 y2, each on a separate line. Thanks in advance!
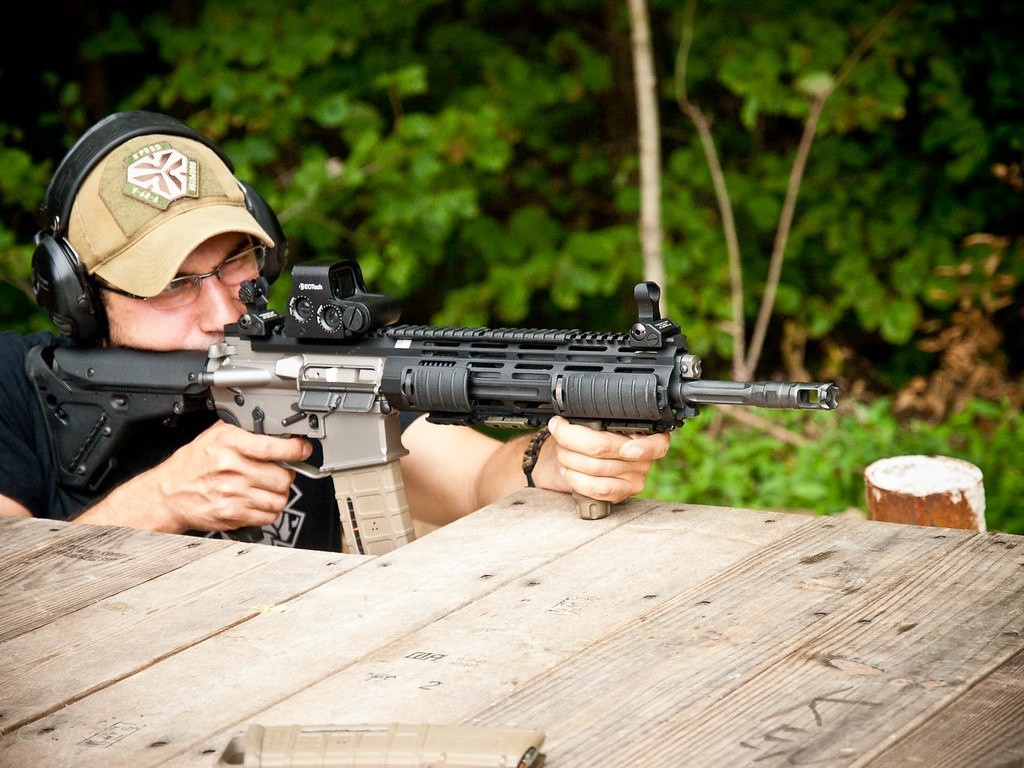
25 258 839 555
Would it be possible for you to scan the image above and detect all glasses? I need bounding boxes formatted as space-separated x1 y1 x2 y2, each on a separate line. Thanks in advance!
92 245 269 310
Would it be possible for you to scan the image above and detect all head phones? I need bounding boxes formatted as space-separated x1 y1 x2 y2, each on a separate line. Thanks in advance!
30 109 289 350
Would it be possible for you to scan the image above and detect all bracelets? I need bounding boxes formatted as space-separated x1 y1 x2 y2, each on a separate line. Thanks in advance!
521 427 551 487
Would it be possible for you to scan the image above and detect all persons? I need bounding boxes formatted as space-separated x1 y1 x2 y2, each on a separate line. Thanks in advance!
1 111 669 556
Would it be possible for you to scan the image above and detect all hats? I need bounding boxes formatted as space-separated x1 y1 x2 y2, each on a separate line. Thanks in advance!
49 110 275 297
